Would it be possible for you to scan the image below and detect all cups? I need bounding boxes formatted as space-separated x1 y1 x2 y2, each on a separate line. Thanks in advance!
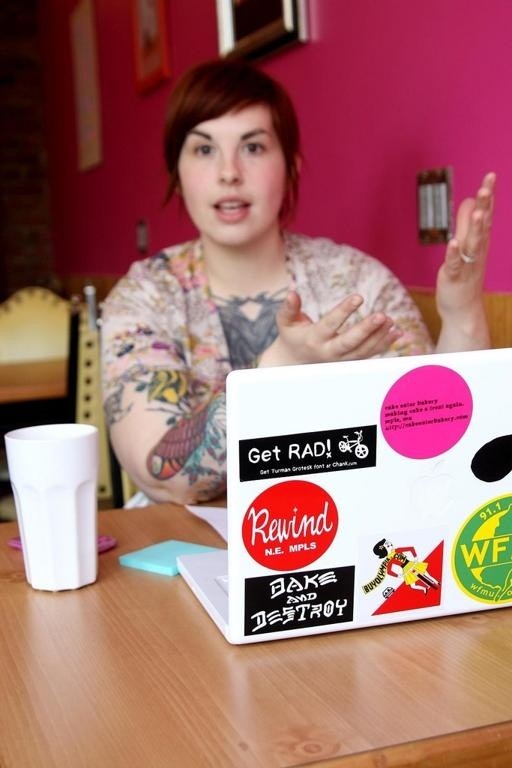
5 422 100 590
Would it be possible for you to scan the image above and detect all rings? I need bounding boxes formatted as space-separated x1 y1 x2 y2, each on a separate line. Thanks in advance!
462 255 475 265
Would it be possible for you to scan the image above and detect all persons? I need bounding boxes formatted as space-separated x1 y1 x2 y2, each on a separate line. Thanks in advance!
102 58 496 508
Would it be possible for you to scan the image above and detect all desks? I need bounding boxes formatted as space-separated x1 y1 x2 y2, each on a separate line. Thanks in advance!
0 494 512 765
1 355 72 406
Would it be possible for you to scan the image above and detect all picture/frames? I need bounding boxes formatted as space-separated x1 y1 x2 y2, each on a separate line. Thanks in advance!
129 0 171 98
213 0 311 64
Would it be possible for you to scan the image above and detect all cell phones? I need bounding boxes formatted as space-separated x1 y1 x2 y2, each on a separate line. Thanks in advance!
9 535 115 555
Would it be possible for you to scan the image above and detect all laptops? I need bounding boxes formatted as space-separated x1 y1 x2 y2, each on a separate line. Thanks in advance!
175 347 512 644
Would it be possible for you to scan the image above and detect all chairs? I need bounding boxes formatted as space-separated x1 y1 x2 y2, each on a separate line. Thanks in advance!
1 280 74 366
107 440 145 510
